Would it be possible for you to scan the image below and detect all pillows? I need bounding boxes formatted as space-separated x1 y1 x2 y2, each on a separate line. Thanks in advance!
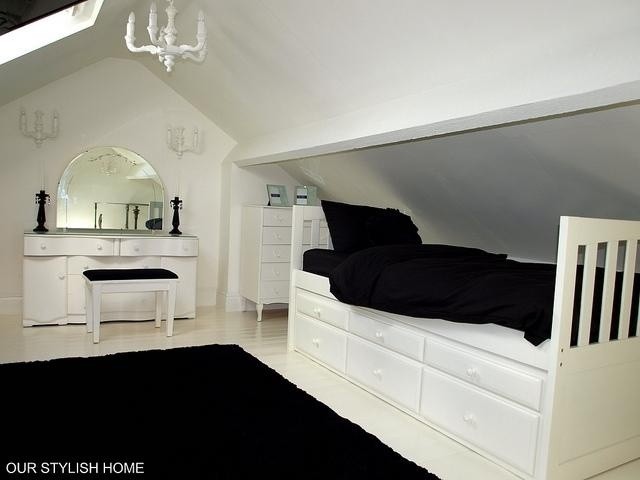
319 198 423 254
403 241 509 263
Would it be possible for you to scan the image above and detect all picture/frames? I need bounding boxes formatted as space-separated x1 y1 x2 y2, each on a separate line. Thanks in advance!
266 182 290 207
293 184 318 206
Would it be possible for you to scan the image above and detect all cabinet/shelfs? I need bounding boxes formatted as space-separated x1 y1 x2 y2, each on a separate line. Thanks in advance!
238 201 332 324
19 231 198 329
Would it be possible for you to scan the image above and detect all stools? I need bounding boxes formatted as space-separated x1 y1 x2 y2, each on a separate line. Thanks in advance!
80 266 181 346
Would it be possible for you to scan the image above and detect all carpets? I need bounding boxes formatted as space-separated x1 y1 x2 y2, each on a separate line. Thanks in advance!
0 342 443 480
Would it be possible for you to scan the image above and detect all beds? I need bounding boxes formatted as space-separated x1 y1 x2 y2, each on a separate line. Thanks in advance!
284 201 640 480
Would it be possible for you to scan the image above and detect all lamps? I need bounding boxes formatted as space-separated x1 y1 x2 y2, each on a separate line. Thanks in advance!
166 125 202 159
123 0 211 73
18 107 60 148
33 190 51 232
169 197 184 236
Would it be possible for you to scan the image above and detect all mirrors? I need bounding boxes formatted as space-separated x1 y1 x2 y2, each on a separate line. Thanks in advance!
56 146 165 231
